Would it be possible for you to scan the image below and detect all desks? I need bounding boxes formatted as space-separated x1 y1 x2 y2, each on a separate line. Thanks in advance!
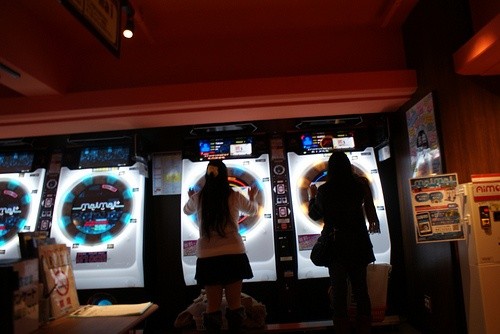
29 301 158 334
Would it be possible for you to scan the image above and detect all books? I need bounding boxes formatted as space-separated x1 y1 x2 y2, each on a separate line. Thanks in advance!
0 231 80 334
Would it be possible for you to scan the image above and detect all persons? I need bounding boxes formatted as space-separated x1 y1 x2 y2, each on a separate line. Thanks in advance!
183 160 259 334
308 151 381 334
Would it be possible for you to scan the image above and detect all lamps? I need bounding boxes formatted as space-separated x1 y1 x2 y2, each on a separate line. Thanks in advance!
123 3 135 39
